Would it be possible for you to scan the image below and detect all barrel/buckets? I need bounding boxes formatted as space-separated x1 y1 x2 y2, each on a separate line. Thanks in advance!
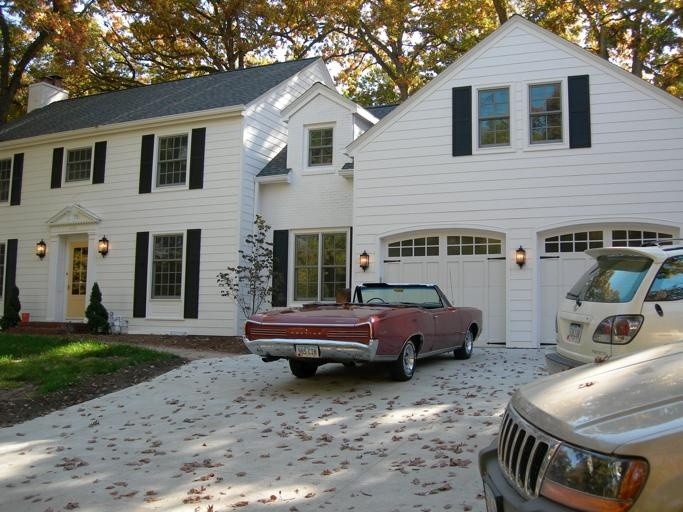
22 312 29 322
112 315 128 334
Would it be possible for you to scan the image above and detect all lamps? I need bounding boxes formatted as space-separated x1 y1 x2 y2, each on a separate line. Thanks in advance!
35 239 46 258
97 235 109 258
360 250 370 272
515 245 527 270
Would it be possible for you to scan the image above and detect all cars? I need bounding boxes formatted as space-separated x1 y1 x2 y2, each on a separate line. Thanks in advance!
479 341 683 512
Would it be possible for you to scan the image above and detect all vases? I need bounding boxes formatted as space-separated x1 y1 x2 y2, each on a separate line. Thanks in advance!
21 312 29 322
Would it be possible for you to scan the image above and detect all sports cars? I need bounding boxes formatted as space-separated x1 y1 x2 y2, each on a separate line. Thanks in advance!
243 282 482 381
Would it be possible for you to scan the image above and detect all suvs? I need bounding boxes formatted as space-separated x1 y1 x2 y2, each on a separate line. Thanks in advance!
543 237 683 378
